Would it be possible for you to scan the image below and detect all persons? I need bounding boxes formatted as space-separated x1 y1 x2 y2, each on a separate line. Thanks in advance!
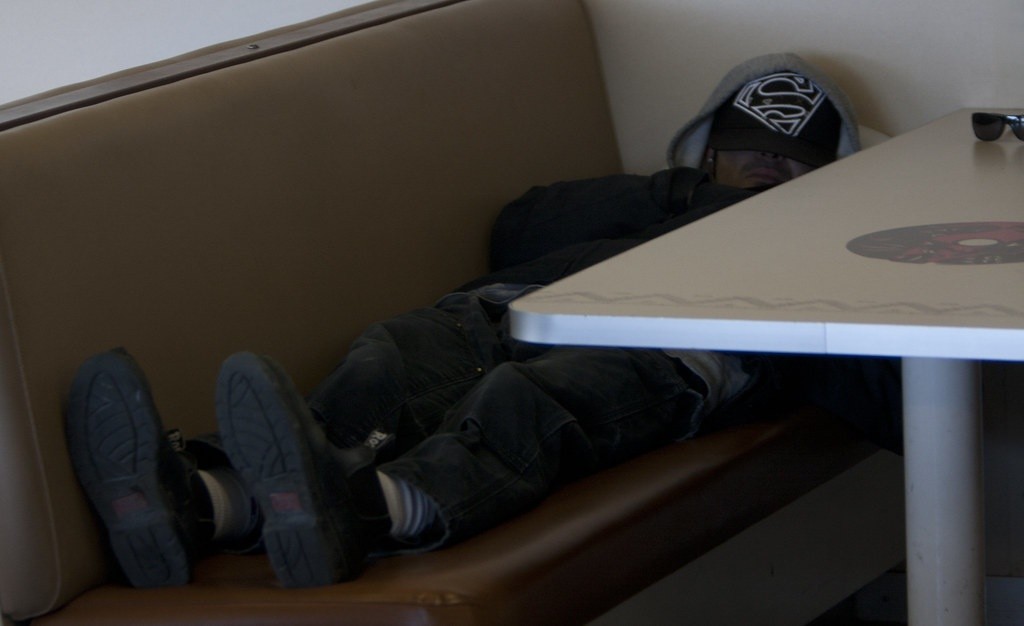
61 53 860 586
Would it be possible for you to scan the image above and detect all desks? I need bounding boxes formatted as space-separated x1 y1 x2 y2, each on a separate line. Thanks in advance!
508 106 1024 626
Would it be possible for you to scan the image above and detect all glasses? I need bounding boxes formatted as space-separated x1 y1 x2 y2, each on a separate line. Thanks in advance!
971 112 1024 143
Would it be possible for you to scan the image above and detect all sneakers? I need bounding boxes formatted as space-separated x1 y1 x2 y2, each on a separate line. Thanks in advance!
62 346 207 591
214 349 379 591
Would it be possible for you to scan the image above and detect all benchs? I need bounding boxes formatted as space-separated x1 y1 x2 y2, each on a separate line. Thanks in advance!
0 0 894 626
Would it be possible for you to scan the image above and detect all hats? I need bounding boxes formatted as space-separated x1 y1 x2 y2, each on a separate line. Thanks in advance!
710 70 843 167
